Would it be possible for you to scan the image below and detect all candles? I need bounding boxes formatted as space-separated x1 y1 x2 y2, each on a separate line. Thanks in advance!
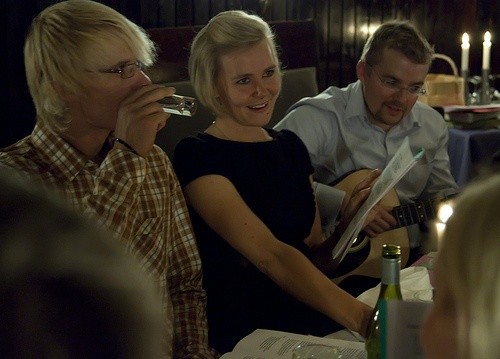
483 31 493 68
461 32 470 71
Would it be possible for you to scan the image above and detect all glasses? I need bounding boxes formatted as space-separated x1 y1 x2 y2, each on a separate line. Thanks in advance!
87 59 140 79
366 61 427 97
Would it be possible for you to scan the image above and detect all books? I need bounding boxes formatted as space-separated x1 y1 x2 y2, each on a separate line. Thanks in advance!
219 328 368 359
431 99 500 131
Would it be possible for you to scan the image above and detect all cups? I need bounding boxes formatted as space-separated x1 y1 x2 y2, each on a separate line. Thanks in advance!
157 94 197 116
292 344 341 359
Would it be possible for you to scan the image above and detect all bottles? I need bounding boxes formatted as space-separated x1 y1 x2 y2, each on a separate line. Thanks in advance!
365 245 404 359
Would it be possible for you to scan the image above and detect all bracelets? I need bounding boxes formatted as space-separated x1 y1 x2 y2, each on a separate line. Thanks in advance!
113 137 142 157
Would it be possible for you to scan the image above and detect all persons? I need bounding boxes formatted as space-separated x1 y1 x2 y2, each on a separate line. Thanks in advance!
273 24 461 269
0 0 215 359
170 11 377 355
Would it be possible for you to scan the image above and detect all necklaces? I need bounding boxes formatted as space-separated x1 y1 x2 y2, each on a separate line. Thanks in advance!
211 116 270 142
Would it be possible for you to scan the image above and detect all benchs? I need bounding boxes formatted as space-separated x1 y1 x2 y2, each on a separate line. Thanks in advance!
135 19 328 157
